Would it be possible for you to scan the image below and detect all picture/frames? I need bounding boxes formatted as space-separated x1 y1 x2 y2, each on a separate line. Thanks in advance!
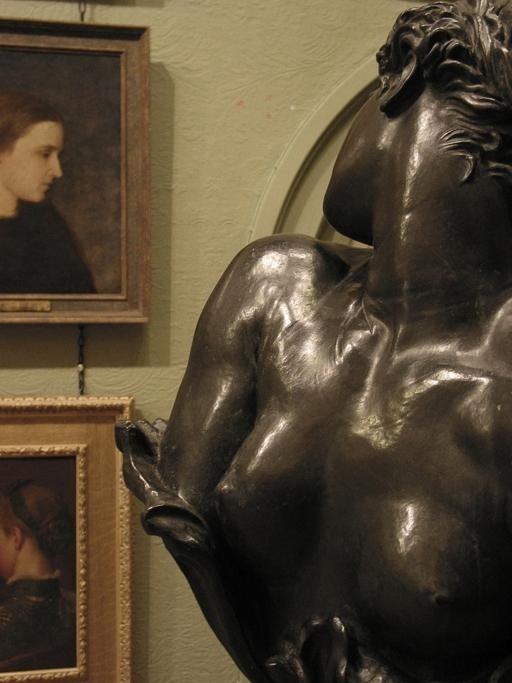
1 396 135 683
0 18 152 325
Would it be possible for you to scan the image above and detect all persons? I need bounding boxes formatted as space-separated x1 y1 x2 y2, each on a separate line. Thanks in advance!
0 480 77 667
0 87 100 294
116 0 512 683
